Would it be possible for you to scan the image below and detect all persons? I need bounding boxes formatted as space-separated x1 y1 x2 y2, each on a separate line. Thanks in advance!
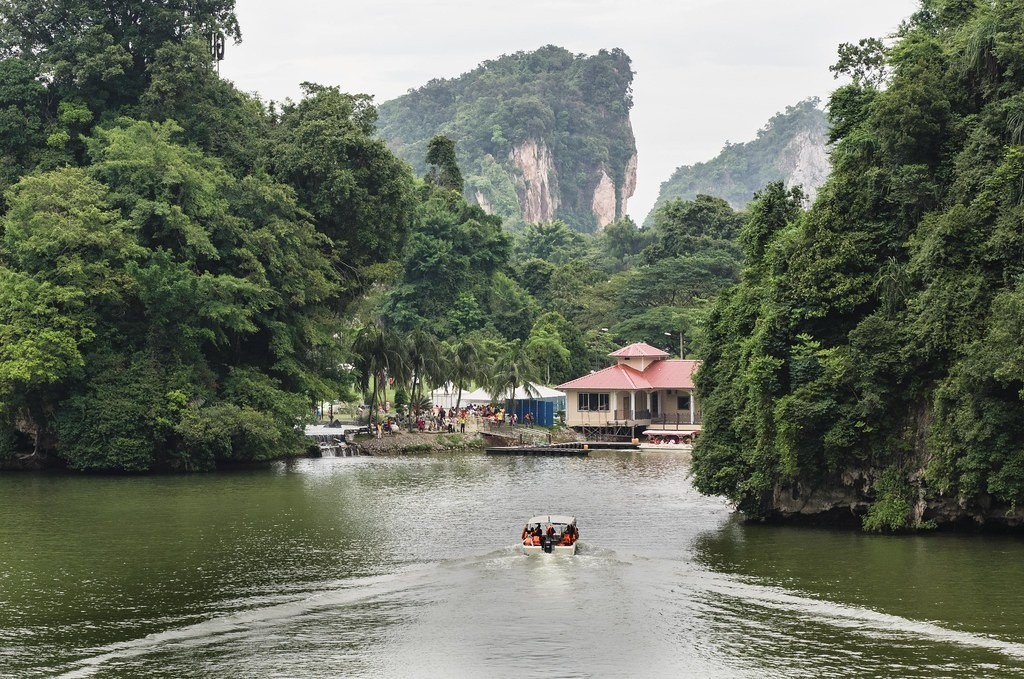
315 403 533 439
521 525 570 540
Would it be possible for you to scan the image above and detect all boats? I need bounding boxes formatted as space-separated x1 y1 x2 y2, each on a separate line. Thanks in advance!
519 515 580 556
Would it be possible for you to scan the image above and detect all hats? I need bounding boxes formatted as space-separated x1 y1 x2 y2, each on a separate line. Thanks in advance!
527 535 531 538
565 531 569 534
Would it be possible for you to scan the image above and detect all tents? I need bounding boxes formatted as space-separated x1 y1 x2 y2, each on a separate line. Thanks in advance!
459 385 505 404
504 381 568 423
426 380 470 410
